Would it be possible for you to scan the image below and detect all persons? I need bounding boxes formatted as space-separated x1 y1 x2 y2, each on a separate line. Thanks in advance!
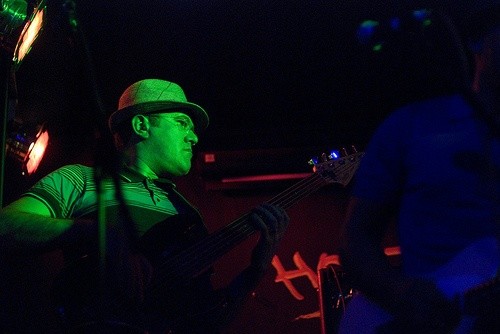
1 80 290 333
337 2 500 333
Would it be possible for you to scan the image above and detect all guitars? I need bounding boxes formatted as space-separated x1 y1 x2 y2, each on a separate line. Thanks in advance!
58 145 363 334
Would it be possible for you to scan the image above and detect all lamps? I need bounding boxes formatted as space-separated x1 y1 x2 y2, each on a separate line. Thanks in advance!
5 118 52 180
0 0 48 71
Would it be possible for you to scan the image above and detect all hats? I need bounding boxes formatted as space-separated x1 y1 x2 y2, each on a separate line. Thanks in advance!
108 79 209 134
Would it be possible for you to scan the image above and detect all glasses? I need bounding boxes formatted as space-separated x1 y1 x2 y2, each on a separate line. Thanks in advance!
147 115 196 133
